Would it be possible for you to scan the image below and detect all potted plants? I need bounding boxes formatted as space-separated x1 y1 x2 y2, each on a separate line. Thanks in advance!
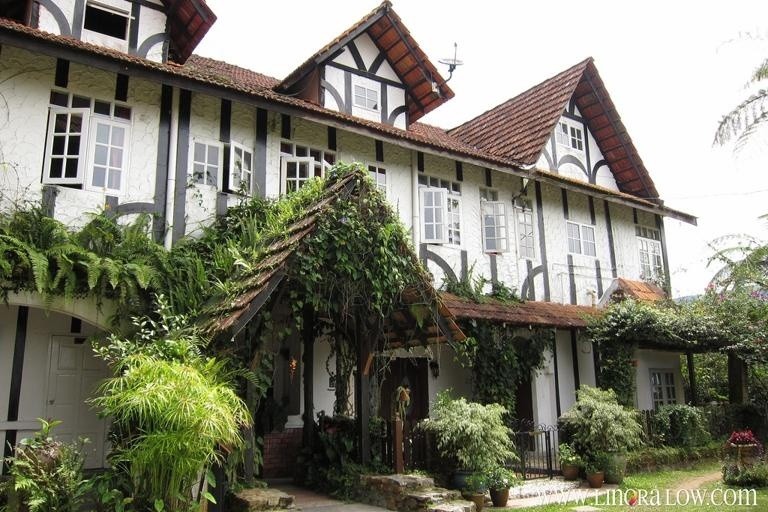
558 386 637 489
424 400 516 512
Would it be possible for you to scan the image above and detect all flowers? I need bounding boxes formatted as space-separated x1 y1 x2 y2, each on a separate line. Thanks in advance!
730 430 759 443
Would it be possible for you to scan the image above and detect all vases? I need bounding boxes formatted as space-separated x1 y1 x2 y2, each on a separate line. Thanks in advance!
727 439 759 458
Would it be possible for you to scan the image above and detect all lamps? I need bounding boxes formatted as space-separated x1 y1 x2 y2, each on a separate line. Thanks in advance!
430 360 440 378
